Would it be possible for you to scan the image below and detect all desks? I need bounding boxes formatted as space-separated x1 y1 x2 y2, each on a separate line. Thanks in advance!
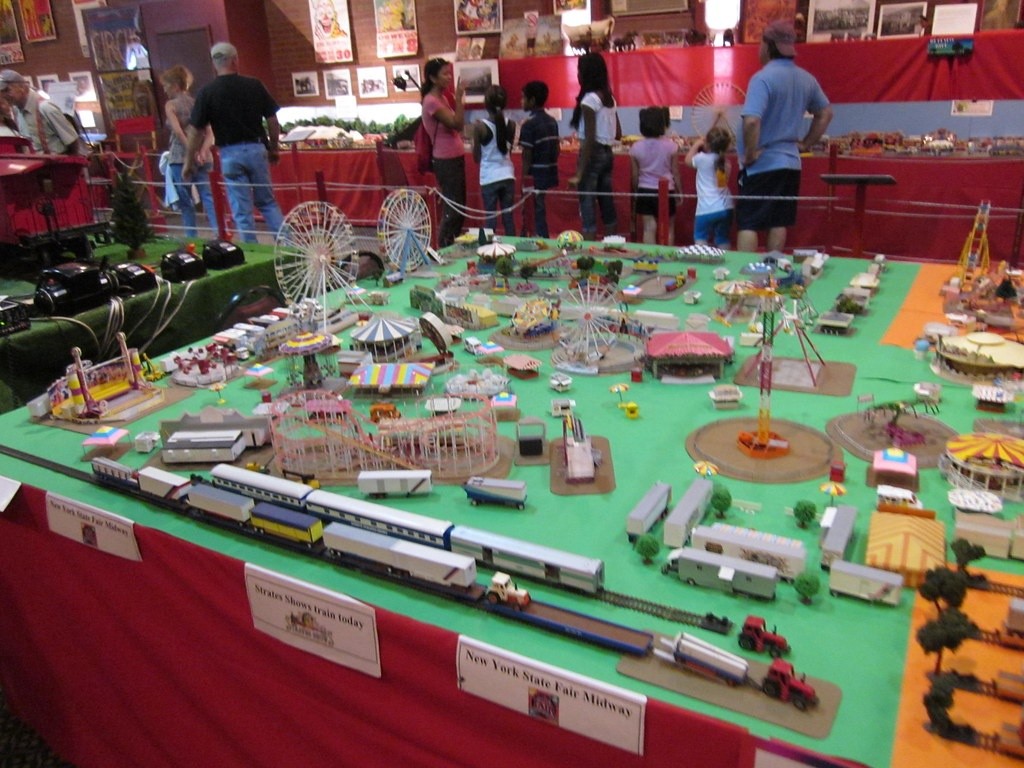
0 143 1024 768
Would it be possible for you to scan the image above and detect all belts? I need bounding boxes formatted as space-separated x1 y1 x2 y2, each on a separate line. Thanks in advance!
220 137 266 147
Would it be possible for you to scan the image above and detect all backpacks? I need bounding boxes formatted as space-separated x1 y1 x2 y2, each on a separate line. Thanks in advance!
414 117 440 175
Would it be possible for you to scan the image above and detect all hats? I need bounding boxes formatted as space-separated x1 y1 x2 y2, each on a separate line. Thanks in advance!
0 70 19 90
764 20 797 57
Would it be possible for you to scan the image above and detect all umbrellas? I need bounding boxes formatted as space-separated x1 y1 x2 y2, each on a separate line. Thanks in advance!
818 480 847 506
609 383 630 402
694 461 720 481
208 382 227 401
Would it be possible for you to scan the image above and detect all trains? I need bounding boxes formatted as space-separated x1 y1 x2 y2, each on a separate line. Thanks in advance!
137 462 604 614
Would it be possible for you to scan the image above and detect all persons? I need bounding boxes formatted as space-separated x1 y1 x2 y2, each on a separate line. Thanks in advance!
408 53 680 251
0 70 83 157
160 65 220 239
180 41 293 246
685 19 833 254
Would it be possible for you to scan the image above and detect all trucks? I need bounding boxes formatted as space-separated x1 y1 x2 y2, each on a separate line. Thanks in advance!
666 546 779 602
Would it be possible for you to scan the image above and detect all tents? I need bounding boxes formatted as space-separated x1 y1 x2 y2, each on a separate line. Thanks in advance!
930 330 1024 409
278 314 434 398
872 430 1024 516
243 363 290 417
453 231 515 271
713 279 756 319
676 244 724 264
81 425 131 462
621 284 642 304
344 285 367 304
476 341 540 418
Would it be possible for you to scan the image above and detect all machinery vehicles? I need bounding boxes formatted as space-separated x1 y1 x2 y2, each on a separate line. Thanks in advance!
758 656 820 711
738 615 790 660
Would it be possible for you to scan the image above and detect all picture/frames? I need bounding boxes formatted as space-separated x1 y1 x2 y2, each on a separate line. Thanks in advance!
876 1 928 39
453 0 503 35
19 0 57 43
980 1 1022 30
357 66 388 98
806 0 876 42
0 0 25 65
12 75 34 118
322 69 352 101
454 58 499 103
37 74 60 92
392 65 420 92
68 72 95 103
612 0 688 16
500 15 562 59
292 71 321 98
741 0 799 44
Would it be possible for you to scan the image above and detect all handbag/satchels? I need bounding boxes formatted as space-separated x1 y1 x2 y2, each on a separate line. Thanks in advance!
40 101 80 140
